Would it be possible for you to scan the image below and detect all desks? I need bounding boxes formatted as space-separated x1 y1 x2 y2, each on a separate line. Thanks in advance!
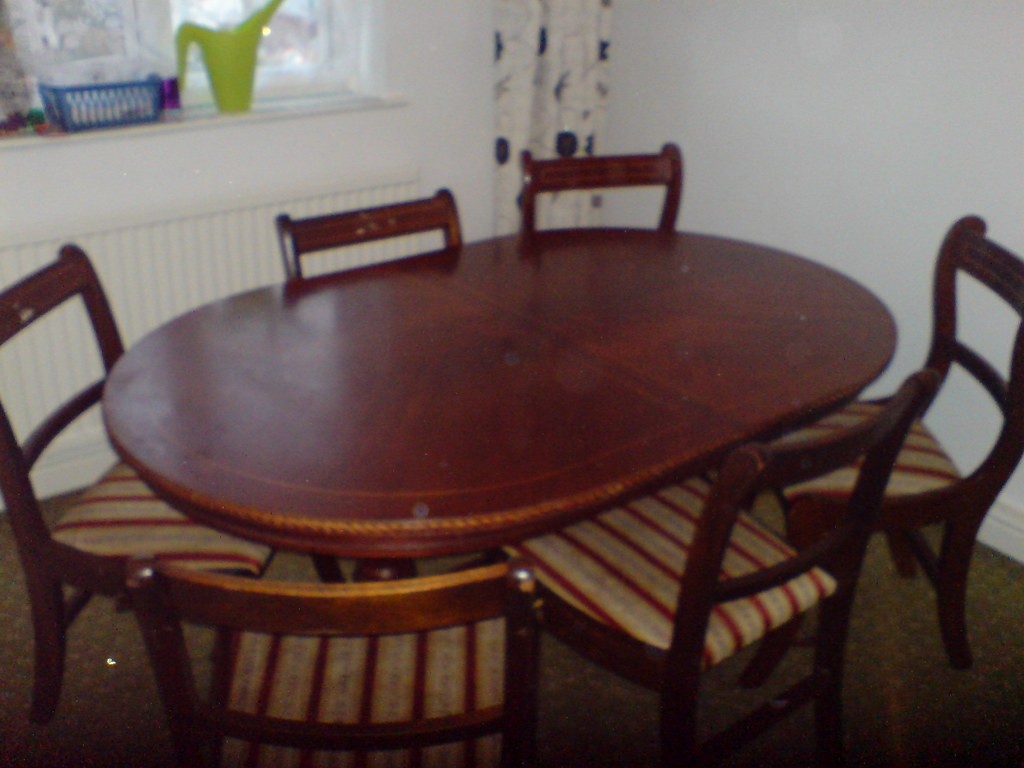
104 225 899 768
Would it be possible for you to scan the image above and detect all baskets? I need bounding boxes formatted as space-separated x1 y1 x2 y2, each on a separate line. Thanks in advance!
37 72 163 132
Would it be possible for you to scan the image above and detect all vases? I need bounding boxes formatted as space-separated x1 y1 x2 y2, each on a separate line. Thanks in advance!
173 0 283 116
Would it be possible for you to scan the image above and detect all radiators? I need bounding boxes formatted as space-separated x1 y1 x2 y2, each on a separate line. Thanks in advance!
0 165 437 471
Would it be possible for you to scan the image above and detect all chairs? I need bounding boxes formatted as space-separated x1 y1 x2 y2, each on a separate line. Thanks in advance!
274 188 465 279
125 553 545 768
0 243 351 741
739 215 1024 685
515 367 937 768
513 144 686 234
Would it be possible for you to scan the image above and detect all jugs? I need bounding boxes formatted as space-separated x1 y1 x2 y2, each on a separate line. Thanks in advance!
175 0 286 113
